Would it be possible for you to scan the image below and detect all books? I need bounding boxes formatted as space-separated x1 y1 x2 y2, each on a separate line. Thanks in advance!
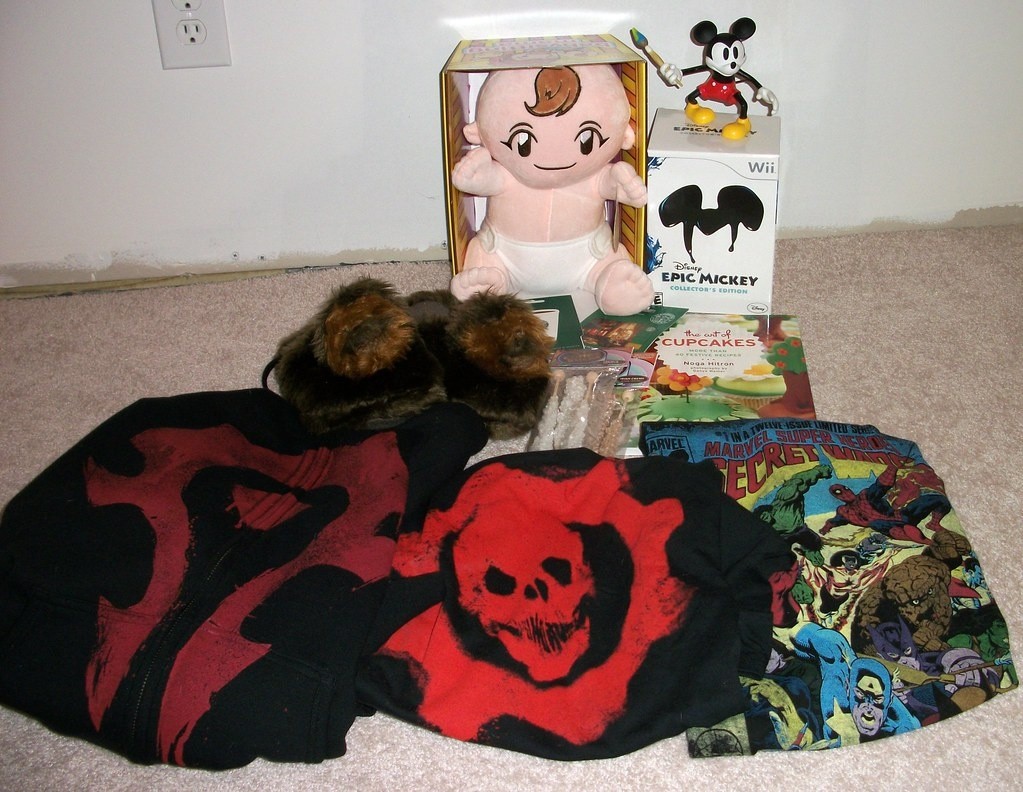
519 295 586 353
580 304 689 353
616 314 817 455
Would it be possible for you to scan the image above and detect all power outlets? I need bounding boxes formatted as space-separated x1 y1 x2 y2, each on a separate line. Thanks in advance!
150 0 232 70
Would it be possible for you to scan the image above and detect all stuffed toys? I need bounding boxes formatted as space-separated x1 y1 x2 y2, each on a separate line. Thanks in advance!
450 63 656 316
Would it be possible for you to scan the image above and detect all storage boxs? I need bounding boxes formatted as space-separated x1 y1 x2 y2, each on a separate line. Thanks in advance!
638 106 780 314
